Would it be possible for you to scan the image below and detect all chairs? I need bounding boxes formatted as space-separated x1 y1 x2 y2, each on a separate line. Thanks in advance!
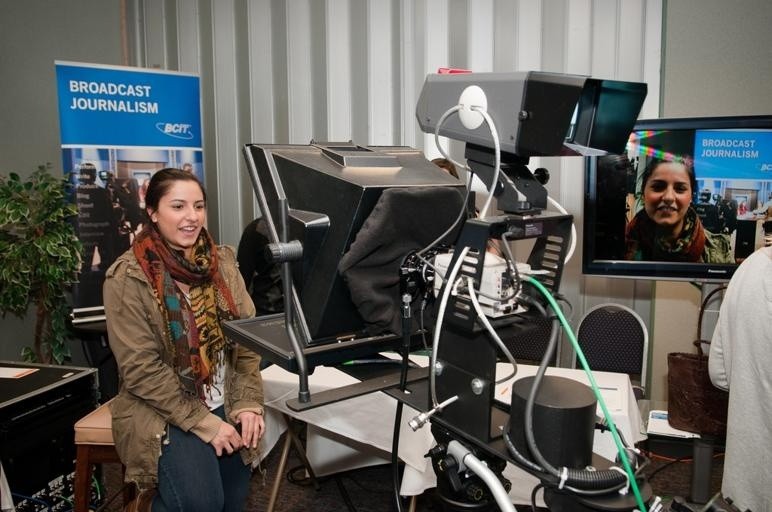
571 303 649 401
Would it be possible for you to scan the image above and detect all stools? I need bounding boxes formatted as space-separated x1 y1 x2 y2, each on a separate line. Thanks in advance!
73 396 121 512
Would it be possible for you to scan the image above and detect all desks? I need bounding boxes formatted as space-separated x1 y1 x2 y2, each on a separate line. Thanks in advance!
253 353 649 512
638 399 726 504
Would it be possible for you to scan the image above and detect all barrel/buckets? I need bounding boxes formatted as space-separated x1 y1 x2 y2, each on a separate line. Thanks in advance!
666 350 729 436
635 438 722 495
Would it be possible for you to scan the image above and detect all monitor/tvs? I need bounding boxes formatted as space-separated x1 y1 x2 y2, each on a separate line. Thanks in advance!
582 115 772 283
241 140 470 347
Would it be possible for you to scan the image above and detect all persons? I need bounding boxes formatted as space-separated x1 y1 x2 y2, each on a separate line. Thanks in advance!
183 164 193 173
103 167 267 493
136 178 150 204
707 206 769 511
625 155 736 263
696 187 719 231
737 200 749 217
753 192 771 217
76 161 114 268
719 186 737 234
